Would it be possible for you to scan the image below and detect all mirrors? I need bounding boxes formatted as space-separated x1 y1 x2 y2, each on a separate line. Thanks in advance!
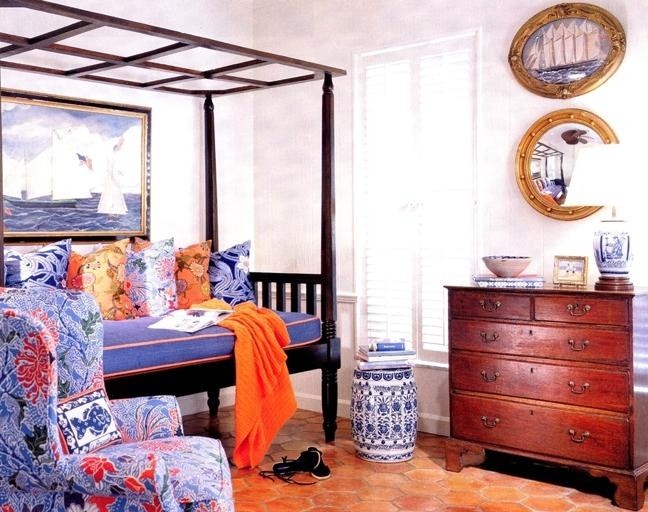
514 107 620 222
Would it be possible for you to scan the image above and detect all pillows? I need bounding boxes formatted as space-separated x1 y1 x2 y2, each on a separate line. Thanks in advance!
57 386 125 454
124 237 178 318
134 236 212 308
3 238 72 290
207 239 256 306
67 237 140 321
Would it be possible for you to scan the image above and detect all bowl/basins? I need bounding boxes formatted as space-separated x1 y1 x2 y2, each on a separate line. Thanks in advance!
482 256 533 277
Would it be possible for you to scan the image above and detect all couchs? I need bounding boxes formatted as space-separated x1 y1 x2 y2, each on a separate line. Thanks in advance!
0 287 235 512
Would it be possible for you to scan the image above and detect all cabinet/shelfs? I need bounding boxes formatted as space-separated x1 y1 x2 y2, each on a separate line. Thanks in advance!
443 283 648 512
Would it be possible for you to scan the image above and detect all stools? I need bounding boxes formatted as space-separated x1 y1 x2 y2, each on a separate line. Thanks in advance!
350 367 419 463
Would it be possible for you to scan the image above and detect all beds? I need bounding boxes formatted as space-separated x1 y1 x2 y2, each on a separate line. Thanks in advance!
1 0 347 443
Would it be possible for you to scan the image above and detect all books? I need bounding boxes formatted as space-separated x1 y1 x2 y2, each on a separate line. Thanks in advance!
355 341 418 371
148 307 234 333
471 273 546 289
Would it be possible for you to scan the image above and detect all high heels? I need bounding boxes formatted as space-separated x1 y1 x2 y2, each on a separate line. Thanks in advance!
259 450 321 485
308 446 331 479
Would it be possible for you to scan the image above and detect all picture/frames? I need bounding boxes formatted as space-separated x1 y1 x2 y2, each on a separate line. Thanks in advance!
553 256 589 286
508 2 627 100
1 87 152 246
